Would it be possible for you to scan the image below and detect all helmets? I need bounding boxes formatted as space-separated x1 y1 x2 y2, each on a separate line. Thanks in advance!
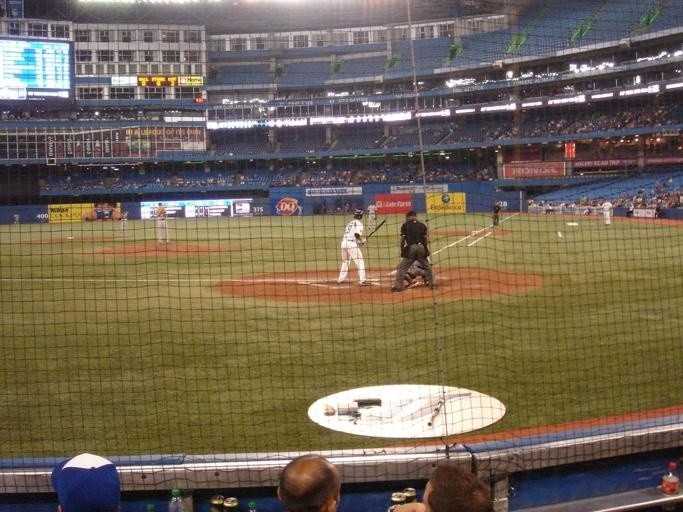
355 209 364 215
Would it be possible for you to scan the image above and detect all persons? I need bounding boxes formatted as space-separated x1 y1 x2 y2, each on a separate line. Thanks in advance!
366 201 377 232
391 210 437 292
277 453 343 512
155 203 169 246
337 210 372 286
396 463 493 512
400 239 431 288
602 199 613 226
120 210 128 232
0 47 683 224
492 202 503 226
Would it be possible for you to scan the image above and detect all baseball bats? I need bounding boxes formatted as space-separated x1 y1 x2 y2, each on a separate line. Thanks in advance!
362 220 386 244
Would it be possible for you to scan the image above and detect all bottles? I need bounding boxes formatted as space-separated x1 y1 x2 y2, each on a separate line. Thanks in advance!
167 488 184 512
660 461 680 511
246 501 256 512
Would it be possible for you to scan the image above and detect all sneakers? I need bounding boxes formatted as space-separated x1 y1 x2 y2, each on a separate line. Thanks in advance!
391 280 431 291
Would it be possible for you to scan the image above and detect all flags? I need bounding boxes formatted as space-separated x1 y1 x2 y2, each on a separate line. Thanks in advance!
639 5 660 26
498 29 528 58
568 16 595 43
440 41 464 65
